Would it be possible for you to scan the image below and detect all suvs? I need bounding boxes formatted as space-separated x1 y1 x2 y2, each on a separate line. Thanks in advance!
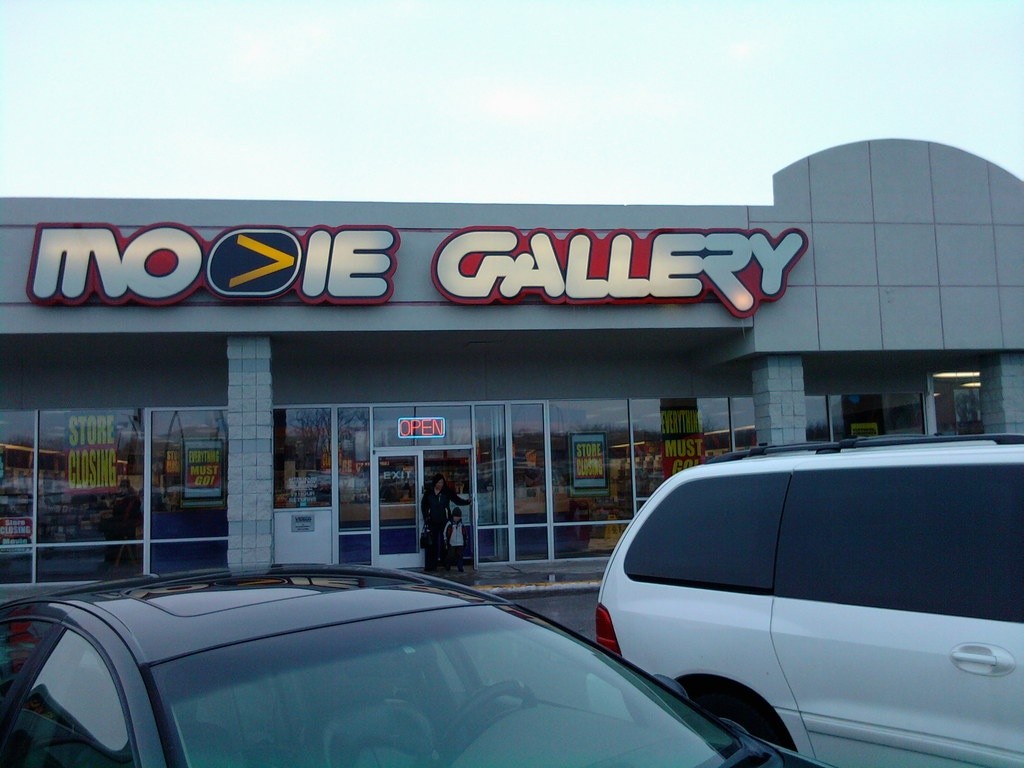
591 429 1024 768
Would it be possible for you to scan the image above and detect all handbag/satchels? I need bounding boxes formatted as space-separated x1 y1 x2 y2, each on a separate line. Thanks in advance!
420 522 432 549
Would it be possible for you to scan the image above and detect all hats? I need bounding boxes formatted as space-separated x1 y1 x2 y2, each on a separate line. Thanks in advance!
452 507 462 517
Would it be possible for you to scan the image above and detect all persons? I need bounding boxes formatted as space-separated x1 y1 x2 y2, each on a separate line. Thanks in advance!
103 479 141 569
421 473 472 572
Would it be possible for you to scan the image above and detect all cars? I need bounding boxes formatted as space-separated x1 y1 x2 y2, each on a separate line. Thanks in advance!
0 562 848 768
